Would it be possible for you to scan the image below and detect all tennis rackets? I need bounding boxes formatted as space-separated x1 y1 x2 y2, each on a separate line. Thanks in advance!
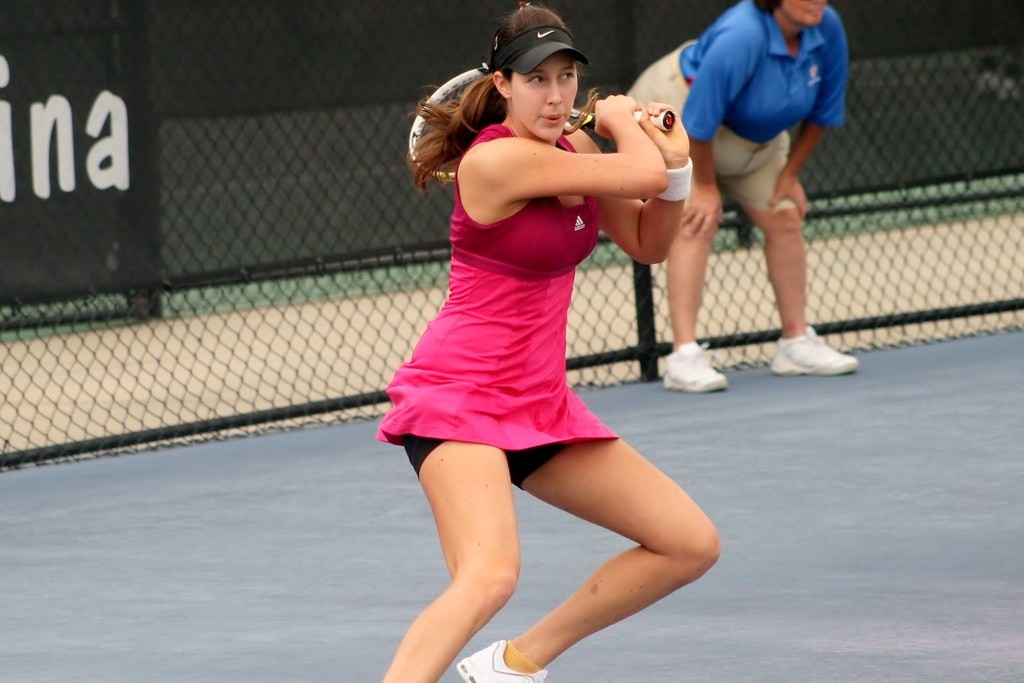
408 64 676 180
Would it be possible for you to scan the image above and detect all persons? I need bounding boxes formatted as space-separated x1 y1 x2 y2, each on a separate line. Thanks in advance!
624 0 860 392
371 3 720 683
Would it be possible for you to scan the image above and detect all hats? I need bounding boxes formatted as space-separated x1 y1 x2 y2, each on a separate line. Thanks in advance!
494 25 589 74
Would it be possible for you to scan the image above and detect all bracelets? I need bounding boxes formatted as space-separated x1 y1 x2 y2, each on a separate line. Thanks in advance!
656 157 693 203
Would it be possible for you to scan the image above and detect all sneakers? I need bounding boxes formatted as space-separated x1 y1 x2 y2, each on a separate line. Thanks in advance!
771 326 857 375
456 639 548 683
662 341 727 393
